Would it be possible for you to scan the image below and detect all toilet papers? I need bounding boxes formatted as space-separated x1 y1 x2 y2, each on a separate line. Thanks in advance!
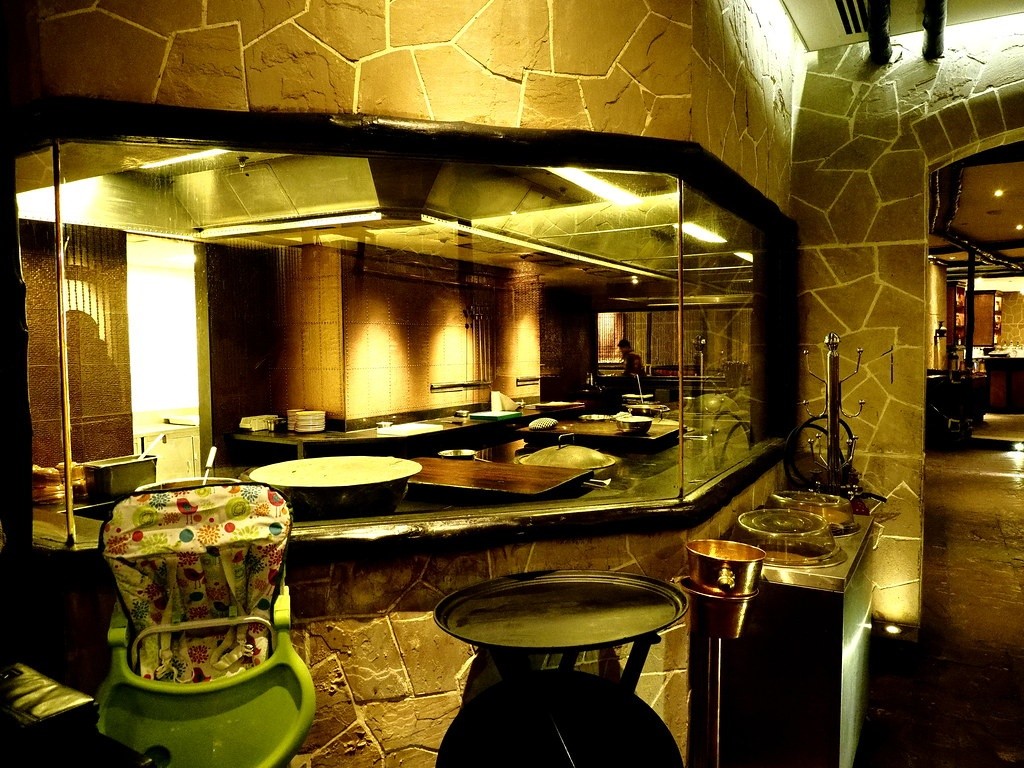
491 391 520 412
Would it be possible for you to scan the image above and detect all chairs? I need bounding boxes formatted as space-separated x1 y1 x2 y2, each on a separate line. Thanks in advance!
91 476 317 768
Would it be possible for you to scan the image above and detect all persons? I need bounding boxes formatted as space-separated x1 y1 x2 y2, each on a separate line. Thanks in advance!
617 339 646 375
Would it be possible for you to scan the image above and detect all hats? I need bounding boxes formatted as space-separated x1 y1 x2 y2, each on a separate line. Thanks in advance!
617 339 630 348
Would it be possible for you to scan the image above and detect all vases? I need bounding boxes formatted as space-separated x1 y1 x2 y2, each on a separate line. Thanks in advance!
937 321 947 336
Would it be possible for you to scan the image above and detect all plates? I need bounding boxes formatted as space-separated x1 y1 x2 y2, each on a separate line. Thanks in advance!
287 409 325 433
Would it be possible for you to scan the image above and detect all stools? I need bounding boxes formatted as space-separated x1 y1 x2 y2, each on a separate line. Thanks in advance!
0 662 99 768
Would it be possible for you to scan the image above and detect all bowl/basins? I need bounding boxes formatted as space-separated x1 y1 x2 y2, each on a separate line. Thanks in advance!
761 492 860 531
579 414 610 423
622 403 671 417
438 450 477 461
456 411 470 417
610 415 652 435
729 508 848 569
685 539 767 596
